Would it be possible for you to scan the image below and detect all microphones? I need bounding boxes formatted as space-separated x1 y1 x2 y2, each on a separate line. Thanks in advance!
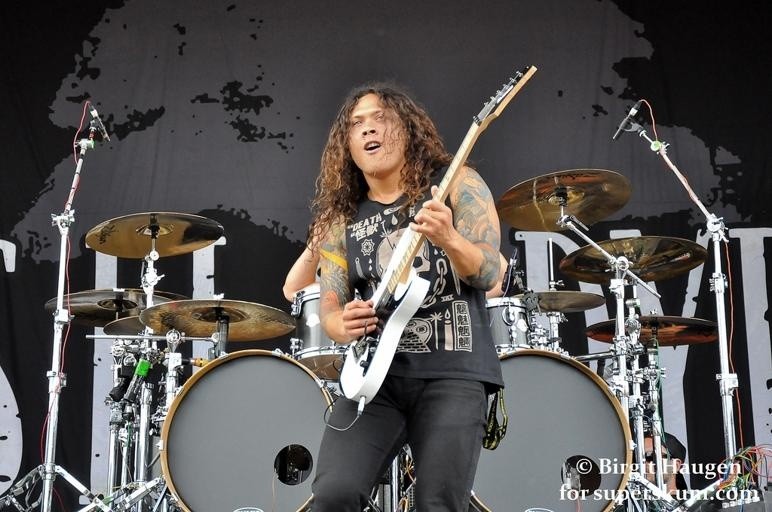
500 249 518 293
612 99 643 142
87 103 110 143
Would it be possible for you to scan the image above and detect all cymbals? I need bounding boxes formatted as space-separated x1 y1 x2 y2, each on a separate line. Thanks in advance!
583 315 719 347
45 288 191 327
513 291 607 312
495 168 629 231
139 300 297 341
85 212 224 259
560 235 709 284
102 315 149 335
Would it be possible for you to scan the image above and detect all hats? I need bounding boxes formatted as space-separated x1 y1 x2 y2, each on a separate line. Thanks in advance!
645 431 688 491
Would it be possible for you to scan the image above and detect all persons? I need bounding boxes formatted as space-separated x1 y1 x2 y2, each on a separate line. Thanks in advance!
309 82 505 512
281 222 508 303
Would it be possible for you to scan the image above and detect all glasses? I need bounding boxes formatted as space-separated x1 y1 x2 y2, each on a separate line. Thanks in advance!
646 451 674 462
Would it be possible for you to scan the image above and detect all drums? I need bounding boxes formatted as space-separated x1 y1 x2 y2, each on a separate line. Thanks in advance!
484 298 536 356
158 349 380 512
469 350 635 511
291 282 352 381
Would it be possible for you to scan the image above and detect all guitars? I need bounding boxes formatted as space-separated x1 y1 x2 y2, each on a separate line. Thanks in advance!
338 65 538 404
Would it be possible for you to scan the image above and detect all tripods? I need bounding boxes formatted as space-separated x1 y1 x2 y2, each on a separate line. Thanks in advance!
0 213 184 512
606 264 680 512
666 219 753 512
